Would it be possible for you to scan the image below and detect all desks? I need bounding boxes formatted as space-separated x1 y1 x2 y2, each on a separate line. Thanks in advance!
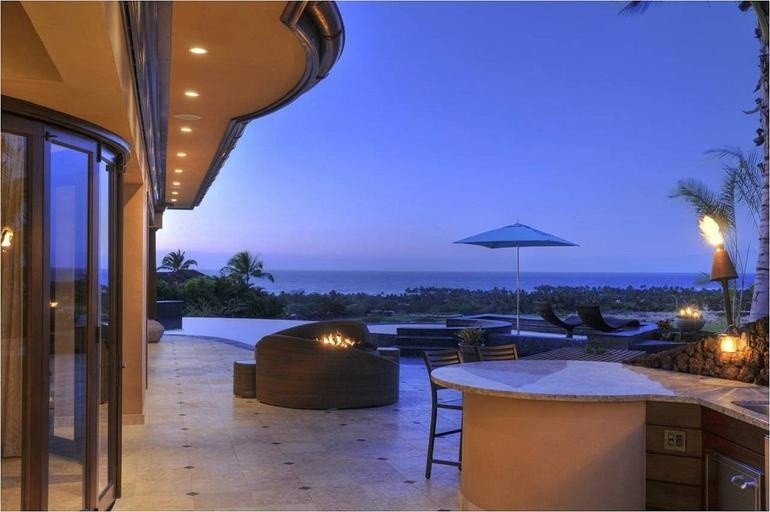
517 346 648 364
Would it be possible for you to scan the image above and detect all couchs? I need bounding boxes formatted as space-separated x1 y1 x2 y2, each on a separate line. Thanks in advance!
254 320 400 411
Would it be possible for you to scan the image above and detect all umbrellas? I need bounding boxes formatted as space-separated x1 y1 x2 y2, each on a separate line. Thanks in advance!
453 221 581 331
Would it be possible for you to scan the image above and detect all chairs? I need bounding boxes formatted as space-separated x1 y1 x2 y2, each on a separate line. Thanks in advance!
421 343 518 479
531 299 641 338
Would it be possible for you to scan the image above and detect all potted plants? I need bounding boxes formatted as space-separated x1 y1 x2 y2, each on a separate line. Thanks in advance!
454 325 489 351
